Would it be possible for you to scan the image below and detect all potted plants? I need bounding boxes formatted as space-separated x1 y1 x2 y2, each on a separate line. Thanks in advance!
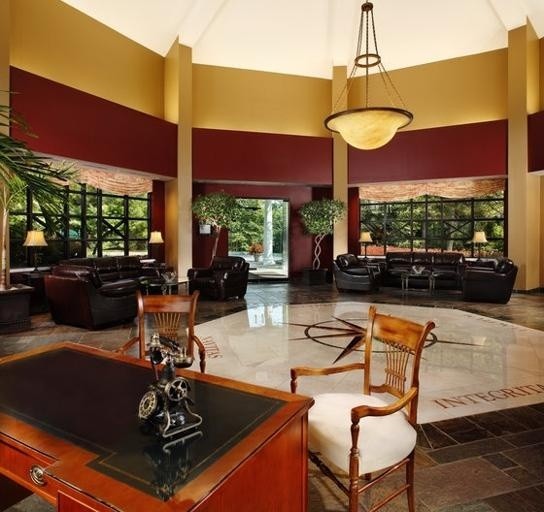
0 88 90 335
189 193 212 234
297 197 347 286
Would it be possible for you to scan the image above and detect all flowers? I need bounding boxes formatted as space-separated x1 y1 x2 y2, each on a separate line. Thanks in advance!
246 241 265 254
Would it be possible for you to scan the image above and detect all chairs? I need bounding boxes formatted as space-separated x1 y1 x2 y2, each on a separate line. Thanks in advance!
290 306 436 512
112 290 205 375
187 255 250 303
462 257 518 304
43 264 139 331
331 253 381 292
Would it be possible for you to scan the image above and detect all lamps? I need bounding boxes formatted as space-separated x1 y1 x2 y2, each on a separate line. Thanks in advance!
149 230 164 267
22 230 48 273
323 0 413 152
471 231 488 261
358 231 373 260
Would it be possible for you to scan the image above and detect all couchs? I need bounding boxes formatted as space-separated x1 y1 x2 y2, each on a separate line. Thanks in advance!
59 255 142 282
379 252 465 291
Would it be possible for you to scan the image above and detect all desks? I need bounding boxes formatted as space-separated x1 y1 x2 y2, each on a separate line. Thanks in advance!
13 270 51 316
0 340 315 512
141 264 178 295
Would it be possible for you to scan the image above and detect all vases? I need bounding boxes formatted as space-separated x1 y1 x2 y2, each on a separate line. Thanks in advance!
252 251 263 262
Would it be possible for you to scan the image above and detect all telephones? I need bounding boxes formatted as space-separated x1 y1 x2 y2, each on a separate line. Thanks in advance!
136 332 204 439
